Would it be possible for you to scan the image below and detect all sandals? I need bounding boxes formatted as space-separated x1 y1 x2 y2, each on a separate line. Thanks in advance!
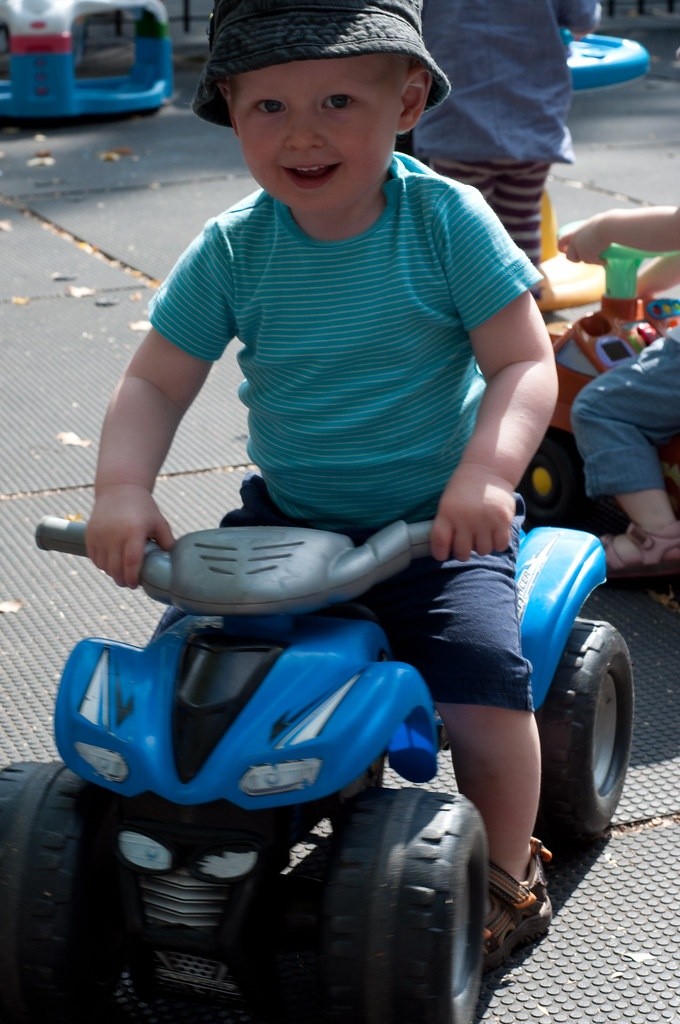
600 521 680 577
482 836 552 969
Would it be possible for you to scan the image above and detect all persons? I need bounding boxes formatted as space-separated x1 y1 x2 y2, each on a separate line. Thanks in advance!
88 0 557 973
558 206 680 579
411 0 602 301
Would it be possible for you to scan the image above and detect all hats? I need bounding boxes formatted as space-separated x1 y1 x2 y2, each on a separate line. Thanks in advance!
192 0 452 128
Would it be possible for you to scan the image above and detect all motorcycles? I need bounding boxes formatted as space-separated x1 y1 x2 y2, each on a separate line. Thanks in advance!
0 493 634 1024
